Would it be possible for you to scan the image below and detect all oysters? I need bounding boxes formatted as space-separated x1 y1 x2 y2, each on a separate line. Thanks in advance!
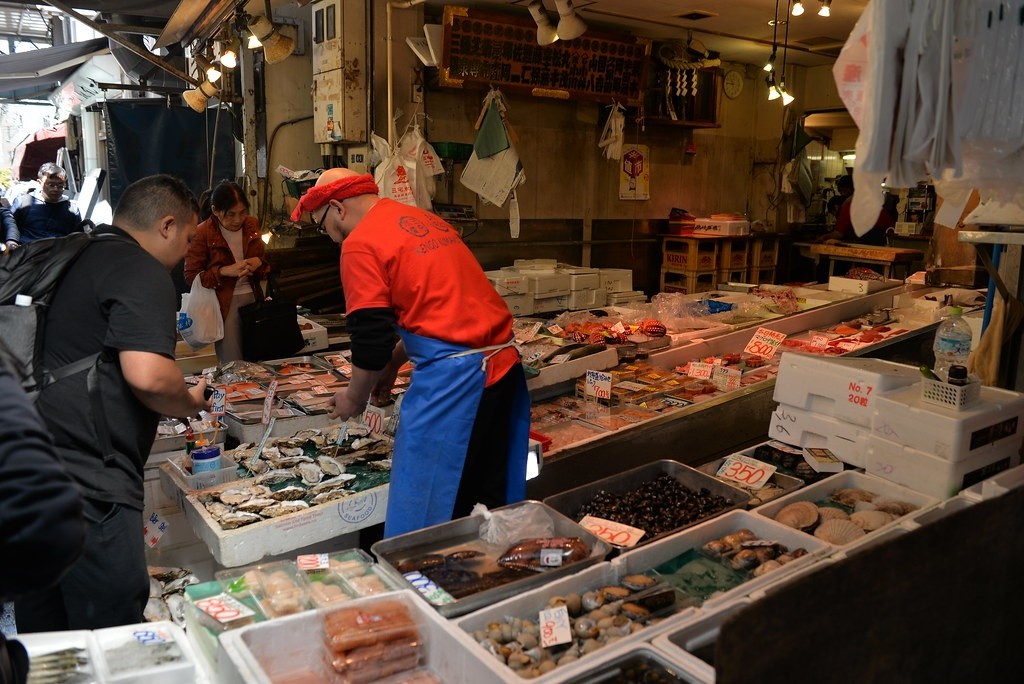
233 497 276 513
141 598 170 621
148 577 163 599
199 423 394 505
163 591 186 631
162 574 200 596
204 501 264 529
147 566 193 582
259 499 310 518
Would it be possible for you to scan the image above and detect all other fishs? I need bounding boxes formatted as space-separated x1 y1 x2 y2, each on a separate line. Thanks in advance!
522 343 589 367
533 343 607 369
26 648 92 684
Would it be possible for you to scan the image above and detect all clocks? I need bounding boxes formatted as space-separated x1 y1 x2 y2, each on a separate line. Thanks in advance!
722 70 744 100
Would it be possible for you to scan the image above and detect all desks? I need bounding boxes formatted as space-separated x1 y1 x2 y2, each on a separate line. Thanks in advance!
828 255 910 278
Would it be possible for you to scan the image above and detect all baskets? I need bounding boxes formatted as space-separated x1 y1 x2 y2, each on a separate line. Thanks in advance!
921 377 981 412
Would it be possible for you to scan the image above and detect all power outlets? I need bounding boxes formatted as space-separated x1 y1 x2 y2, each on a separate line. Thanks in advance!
411 84 424 104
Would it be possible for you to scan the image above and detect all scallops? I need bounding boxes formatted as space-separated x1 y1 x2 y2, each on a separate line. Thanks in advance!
811 507 847 527
813 519 867 545
849 510 893 531
775 501 818 530
876 500 920 521
829 488 874 507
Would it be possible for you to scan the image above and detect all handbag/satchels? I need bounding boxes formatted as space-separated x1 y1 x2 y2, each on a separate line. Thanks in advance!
238 263 306 363
178 271 224 353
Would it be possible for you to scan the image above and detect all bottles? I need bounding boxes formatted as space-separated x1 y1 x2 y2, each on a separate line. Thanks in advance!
195 432 207 449
186 434 195 455
934 308 973 384
191 446 222 475
948 366 967 406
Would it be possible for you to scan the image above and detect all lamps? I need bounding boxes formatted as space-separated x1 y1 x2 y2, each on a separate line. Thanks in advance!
554 0 587 41
182 79 219 113
247 13 295 65
528 0 559 46
220 39 240 69
195 54 222 83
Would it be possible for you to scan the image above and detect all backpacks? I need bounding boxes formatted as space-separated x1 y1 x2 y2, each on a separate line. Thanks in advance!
0 231 143 404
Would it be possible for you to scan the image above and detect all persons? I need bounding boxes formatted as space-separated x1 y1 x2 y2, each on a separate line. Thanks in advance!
184 181 265 364
0 161 213 684
807 176 893 282
291 167 531 540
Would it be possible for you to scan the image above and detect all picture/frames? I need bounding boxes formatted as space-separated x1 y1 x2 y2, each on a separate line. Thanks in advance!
272 15 306 56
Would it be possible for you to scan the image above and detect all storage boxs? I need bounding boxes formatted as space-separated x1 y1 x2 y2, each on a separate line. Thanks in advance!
283 169 322 200
284 193 312 224
7 218 1024 684
909 185 936 198
909 198 933 212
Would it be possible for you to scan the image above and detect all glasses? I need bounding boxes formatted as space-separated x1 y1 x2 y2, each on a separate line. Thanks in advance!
317 200 344 235
41 180 66 190
